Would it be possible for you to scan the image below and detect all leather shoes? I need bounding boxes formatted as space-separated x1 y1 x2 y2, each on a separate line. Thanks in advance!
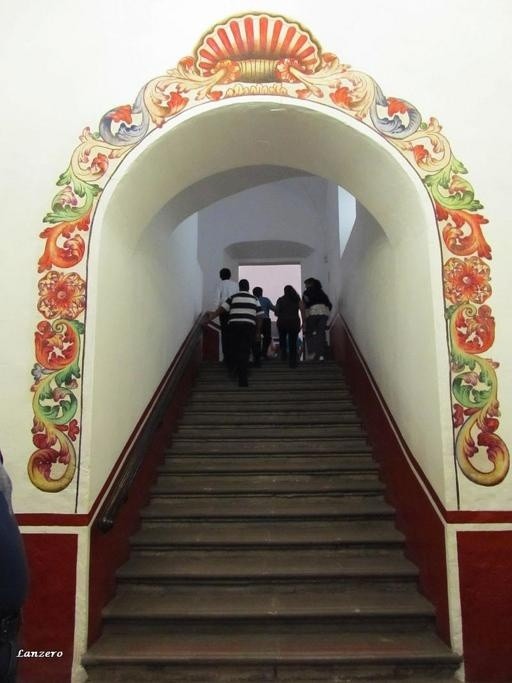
238 368 249 387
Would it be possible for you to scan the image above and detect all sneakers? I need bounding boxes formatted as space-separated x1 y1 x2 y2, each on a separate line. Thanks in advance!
220 356 327 369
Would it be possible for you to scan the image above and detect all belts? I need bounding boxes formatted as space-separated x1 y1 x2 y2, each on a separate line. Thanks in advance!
0 614 23 645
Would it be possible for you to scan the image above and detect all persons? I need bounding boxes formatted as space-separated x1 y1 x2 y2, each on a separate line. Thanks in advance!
1 451 35 680
256 332 304 364
301 276 333 365
202 278 264 387
273 283 305 369
250 284 276 359
213 268 240 368
